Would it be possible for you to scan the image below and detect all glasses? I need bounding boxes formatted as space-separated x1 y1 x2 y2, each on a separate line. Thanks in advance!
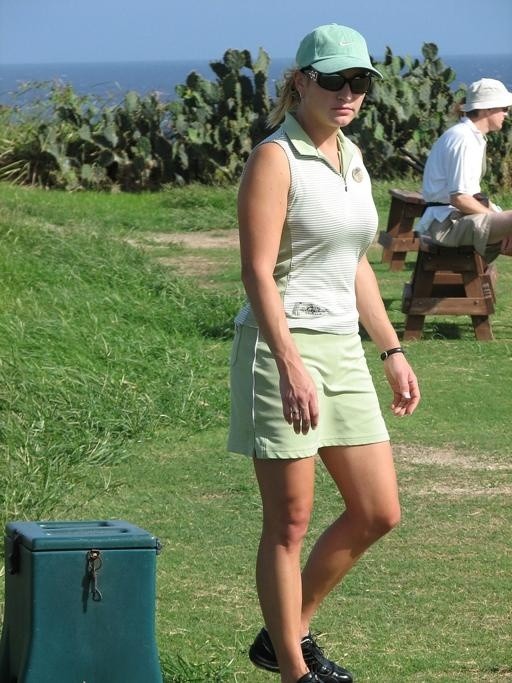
300 69 371 94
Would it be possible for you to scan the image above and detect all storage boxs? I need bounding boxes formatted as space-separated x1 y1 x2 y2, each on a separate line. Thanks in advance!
1 517 162 682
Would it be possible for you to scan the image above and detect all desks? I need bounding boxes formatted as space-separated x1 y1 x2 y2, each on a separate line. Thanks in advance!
375 186 489 272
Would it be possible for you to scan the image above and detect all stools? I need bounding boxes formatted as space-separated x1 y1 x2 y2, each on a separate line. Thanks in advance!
399 236 498 342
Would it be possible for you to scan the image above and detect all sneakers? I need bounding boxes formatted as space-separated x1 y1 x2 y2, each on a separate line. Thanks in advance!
249 627 353 683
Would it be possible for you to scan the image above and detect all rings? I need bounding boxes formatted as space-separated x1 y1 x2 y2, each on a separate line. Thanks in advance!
292 411 299 415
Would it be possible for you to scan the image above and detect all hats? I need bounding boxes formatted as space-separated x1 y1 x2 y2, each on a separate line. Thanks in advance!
458 78 512 113
296 22 383 79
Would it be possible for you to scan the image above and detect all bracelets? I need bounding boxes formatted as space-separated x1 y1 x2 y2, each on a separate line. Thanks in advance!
381 348 404 360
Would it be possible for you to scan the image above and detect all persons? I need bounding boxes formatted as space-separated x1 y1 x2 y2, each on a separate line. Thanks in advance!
225 25 422 683
414 78 512 264
